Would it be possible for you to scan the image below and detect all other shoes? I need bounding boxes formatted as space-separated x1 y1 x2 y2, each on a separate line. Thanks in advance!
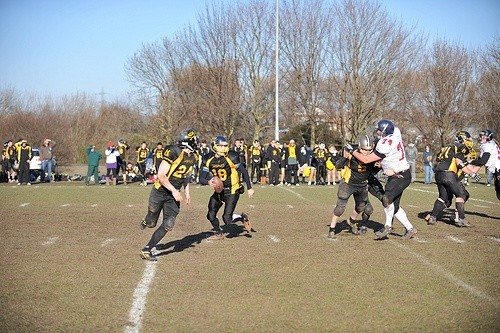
346 218 360 235
139 217 146 230
427 215 438 225
327 229 336 239
373 224 394 239
204 228 228 240
360 224 367 234
241 213 251 234
401 224 417 239
139 249 157 261
451 216 475 228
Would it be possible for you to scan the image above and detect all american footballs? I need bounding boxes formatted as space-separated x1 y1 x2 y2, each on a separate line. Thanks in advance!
212 176 223 193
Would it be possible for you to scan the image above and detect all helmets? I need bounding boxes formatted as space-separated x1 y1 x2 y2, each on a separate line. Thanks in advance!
176 128 198 150
478 129 493 141
211 136 228 151
357 135 375 155
376 120 394 136
456 130 472 143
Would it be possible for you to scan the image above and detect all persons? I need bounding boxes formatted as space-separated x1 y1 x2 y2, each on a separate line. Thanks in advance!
345 119 418 239
423 144 433 185
424 129 500 226
189 138 217 184
104 141 121 185
233 139 339 186
405 141 418 182
85 144 102 185
116 138 131 185
14 140 32 186
1 139 19 183
124 141 167 186
327 133 396 238
39 137 57 182
198 135 256 240
139 129 199 261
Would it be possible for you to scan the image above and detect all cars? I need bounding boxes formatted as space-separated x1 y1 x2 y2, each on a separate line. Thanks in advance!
12 149 42 171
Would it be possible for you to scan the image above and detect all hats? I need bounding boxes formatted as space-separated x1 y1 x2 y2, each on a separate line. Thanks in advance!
118 138 124 143
107 141 113 146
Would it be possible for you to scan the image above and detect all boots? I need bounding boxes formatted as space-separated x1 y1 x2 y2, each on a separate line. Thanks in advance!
105 177 110 186
112 178 117 186
